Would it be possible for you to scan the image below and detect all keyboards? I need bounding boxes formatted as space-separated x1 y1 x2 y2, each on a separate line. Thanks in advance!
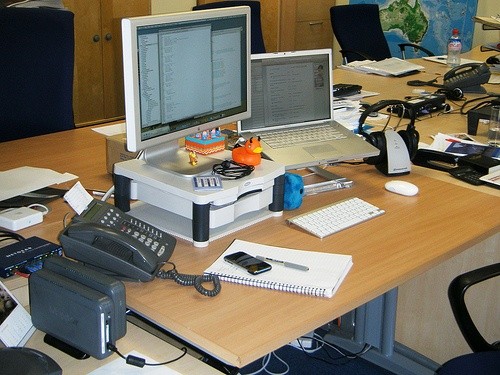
287 196 386 239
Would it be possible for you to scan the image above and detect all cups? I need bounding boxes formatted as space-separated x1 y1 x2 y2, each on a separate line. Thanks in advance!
487 106 500 147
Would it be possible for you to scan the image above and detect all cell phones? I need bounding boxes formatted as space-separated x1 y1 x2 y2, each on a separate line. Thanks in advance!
224 251 272 275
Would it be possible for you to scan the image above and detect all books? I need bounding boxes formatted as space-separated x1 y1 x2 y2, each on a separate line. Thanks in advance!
204 238 354 298
457 153 500 174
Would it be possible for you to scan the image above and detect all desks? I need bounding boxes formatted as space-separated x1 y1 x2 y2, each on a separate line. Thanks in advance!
0 43 500 375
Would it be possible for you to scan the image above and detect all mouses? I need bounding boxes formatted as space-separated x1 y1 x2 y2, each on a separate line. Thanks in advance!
385 180 418 197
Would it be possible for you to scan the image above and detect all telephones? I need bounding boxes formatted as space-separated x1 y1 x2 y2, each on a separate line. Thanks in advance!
444 61 492 94
57 198 177 282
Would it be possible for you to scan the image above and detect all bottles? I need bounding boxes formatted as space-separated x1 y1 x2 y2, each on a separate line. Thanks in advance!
446 29 462 67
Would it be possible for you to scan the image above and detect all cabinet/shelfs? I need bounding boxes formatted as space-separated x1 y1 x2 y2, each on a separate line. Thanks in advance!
62 0 152 127
196 0 333 55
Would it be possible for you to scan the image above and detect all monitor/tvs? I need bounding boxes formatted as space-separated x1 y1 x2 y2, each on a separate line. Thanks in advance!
121 7 252 177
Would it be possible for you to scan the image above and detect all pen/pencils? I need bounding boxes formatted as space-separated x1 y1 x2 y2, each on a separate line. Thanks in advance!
86 189 114 198
256 256 310 271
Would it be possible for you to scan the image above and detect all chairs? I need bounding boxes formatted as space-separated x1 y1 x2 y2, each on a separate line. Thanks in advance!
432 261 500 375
191 0 266 55
329 4 435 65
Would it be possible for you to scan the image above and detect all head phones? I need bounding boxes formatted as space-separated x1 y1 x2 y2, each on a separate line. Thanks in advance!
358 99 420 164
436 87 464 99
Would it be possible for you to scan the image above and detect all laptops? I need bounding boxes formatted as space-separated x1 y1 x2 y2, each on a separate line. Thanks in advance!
236 47 381 173
